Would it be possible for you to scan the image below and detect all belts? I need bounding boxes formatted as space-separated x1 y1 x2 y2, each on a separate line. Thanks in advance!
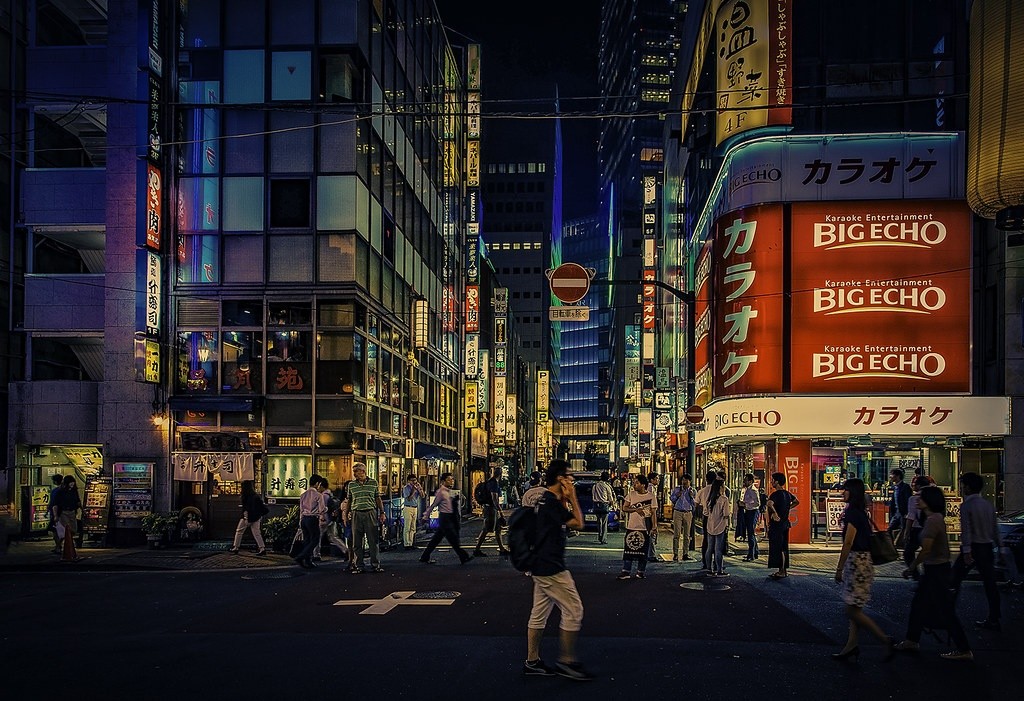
674 508 691 512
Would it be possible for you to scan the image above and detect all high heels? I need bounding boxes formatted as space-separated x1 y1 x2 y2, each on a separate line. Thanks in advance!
885 636 899 664
256 549 266 556
230 549 239 555
831 645 861 661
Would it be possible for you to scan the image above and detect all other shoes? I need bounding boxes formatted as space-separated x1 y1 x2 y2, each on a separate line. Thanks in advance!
397 544 509 562
372 561 384 572
555 660 588 677
525 657 557 675
352 567 361 573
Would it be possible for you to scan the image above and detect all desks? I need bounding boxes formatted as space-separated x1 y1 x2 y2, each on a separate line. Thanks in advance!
811 510 828 541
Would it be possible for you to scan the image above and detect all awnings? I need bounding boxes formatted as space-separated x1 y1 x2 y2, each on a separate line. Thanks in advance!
414 443 461 462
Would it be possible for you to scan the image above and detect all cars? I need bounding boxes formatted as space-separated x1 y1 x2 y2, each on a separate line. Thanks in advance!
994 508 1024 593
572 472 620 532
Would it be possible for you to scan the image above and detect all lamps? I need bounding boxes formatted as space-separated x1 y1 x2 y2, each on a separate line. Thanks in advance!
776 438 790 444
949 449 957 463
410 294 428 351
943 437 965 449
149 398 163 426
813 435 874 449
405 438 414 459
922 436 947 445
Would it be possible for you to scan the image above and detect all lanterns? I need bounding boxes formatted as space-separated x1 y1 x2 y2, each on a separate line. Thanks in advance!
966 0 1024 230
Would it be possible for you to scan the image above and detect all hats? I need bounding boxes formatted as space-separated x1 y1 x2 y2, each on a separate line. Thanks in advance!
353 462 366 470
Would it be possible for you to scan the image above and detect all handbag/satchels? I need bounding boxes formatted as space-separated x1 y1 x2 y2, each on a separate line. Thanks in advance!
289 529 305 558
616 554 793 580
622 528 651 559
868 516 900 565
594 502 609 513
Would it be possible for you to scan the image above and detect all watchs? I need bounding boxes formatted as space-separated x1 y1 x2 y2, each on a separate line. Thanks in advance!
381 512 385 514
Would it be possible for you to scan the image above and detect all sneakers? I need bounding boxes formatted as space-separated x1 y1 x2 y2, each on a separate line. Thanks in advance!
894 640 920 649
941 650 973 659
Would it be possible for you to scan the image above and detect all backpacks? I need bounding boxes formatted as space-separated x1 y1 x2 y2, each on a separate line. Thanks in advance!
474 482 491 503
913 494 929 527
328 496 343 522
509 499 565 577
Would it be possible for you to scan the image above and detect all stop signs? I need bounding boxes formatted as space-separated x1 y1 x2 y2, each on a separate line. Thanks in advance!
549 261 591 304
686 405 705 423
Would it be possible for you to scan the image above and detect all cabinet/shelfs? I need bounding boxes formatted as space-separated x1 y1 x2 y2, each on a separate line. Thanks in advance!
946 522 961 541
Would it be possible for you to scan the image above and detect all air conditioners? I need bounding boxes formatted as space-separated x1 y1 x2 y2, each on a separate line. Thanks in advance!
412 384 426 403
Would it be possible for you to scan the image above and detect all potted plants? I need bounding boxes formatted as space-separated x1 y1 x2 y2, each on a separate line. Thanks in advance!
264 505 301 551
141 509 180 550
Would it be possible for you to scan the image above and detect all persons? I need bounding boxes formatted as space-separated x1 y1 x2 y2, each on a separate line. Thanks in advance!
591 472 616 543
403 474 425 551
612 472 664 563
472 467 511 557
669 473 697 560
767 472 799 578
419 473 475 565
695 470 734 577
893 485 974 659
229 480 267 557
615 474 658 580
951 472 1003 629
294 473 357 572
831 478 894 660
525 459 594 680
735 473 767 562
44 474 87 563
344 463 386 574
507 467 546 507
888 467 938 580
